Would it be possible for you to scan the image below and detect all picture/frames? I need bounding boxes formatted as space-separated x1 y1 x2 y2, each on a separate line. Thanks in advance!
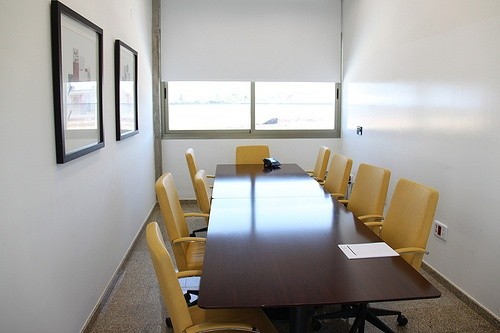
50 0 105 164
114 38 139 142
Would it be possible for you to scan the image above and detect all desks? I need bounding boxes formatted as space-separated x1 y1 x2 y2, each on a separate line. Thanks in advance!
196 162 442 333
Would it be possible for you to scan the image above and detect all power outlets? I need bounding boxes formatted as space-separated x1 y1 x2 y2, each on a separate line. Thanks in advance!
434 220 448 242
349 174 354 185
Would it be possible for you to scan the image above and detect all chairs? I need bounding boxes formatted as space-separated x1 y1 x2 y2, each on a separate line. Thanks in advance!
307 176 440 332
184 147 216 237
236 144 269 165
338 163 391 237
146 221 277 333
304 144 331 182
194 169 210 218
318 153 354 199
156 171 209 271
